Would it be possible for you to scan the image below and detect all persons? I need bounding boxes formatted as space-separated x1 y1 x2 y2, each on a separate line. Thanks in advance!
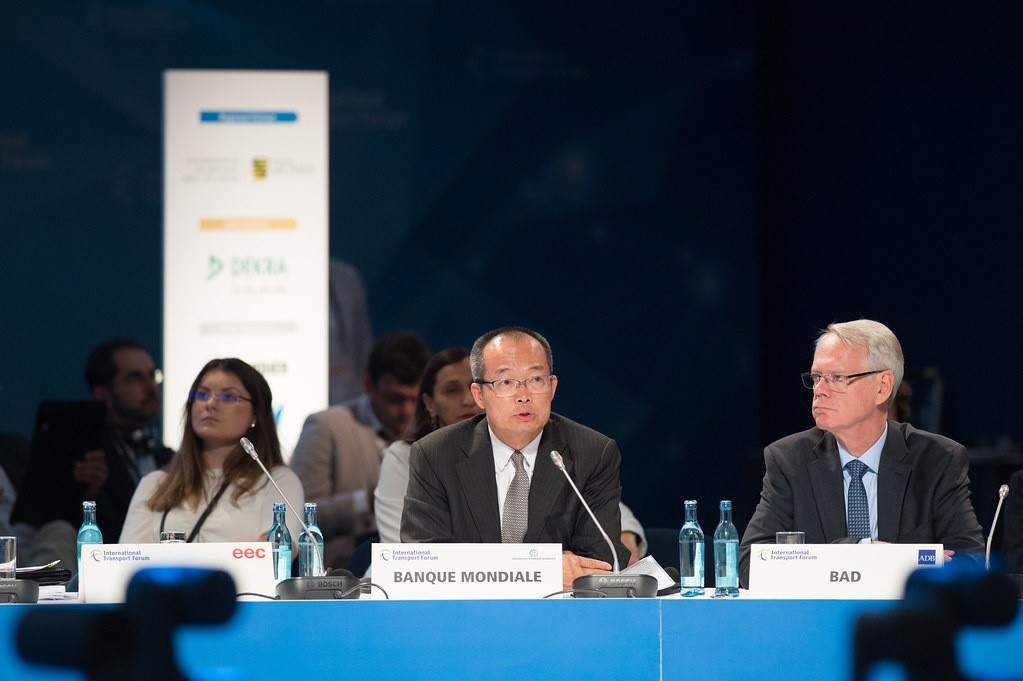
119 358 306 579
738 319 1023 588
0 339 176 585
288 326 647 590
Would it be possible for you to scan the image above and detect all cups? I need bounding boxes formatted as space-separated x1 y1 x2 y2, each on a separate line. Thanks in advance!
776 531 805 544
0 536 17 581
160 531 186 543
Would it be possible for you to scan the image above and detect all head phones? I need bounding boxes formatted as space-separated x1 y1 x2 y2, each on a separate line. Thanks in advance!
97 400 158 453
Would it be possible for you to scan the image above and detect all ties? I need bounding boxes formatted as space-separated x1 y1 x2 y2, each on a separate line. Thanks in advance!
501 452 530 543
845 459 871 539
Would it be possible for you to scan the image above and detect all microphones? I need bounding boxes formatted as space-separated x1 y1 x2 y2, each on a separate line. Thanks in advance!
984 484 1010 569
239 437 361 601
550 450 659 599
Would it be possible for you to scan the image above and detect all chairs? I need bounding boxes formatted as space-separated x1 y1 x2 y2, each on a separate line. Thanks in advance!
643 528 717 582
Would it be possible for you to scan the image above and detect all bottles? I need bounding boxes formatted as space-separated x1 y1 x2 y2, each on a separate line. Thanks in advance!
267 503 292 579
298 503 323 576
713 500 740 597
678 500 705 594
76 500 103 563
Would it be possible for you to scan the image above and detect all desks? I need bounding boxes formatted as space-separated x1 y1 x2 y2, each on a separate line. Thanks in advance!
0 584 1023 681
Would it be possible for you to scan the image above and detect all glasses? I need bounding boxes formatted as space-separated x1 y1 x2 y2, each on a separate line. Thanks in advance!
800 368 889 393
478 375 551 397
125 368 163 384
189 387 252 409
